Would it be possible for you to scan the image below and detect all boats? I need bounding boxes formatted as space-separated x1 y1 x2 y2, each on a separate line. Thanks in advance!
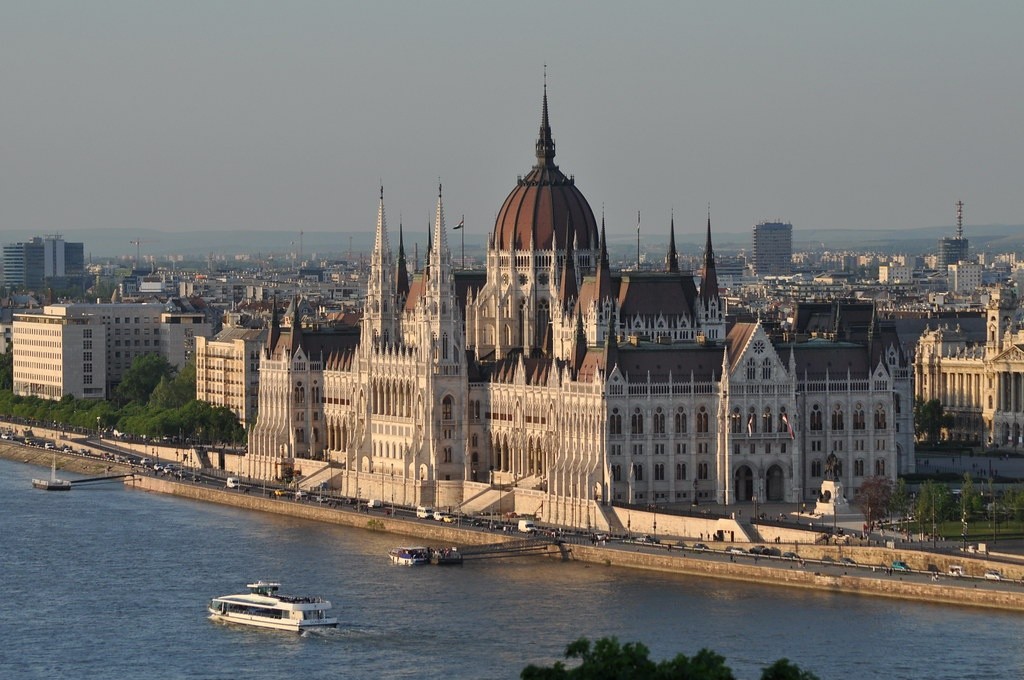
31 451 72 490
207 580 340 633
428 553 463 564
387 546 430 565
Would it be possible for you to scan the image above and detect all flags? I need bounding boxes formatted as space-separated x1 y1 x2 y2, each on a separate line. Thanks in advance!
747 417 753 438
452 219 464 230
782 413 795 440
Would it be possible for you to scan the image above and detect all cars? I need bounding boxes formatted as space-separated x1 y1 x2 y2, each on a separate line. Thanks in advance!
783 552 800 558
693 543 709 549
749 546 772 555
112 430 191 444
637 534 650 542
296 490 307 497
841 558 856 564
444 516 458 523
588 533 607 541
317 497 328 503
273 489 286 496
891 561 910 570
731 547 748 553
434 511 448 521
984 572 1004 582
1 433 188 478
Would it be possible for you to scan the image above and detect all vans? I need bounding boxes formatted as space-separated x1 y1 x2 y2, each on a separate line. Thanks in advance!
518 519 537 534
227 477 239 488
417 507 433 519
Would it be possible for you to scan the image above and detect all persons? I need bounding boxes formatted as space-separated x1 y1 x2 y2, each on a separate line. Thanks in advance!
885 567 893 576
668 543 671 552
931 571 939 581
798 558 806 568
399 545 450 560
